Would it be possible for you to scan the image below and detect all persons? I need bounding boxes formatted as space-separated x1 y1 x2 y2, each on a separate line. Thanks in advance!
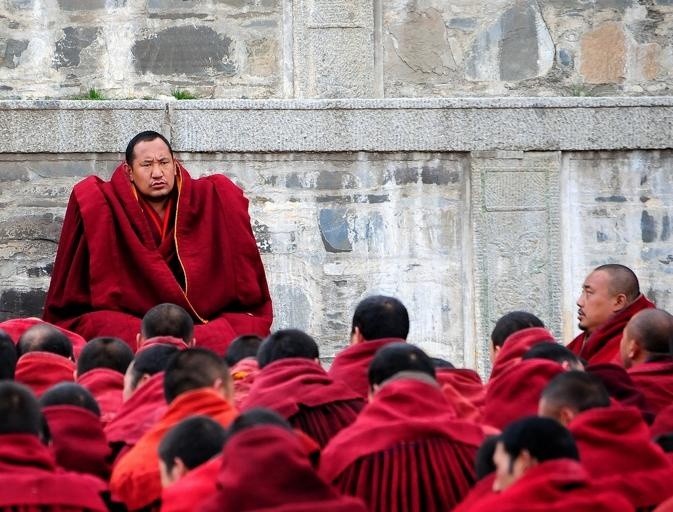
0 264 671 511
39 131 272 356
